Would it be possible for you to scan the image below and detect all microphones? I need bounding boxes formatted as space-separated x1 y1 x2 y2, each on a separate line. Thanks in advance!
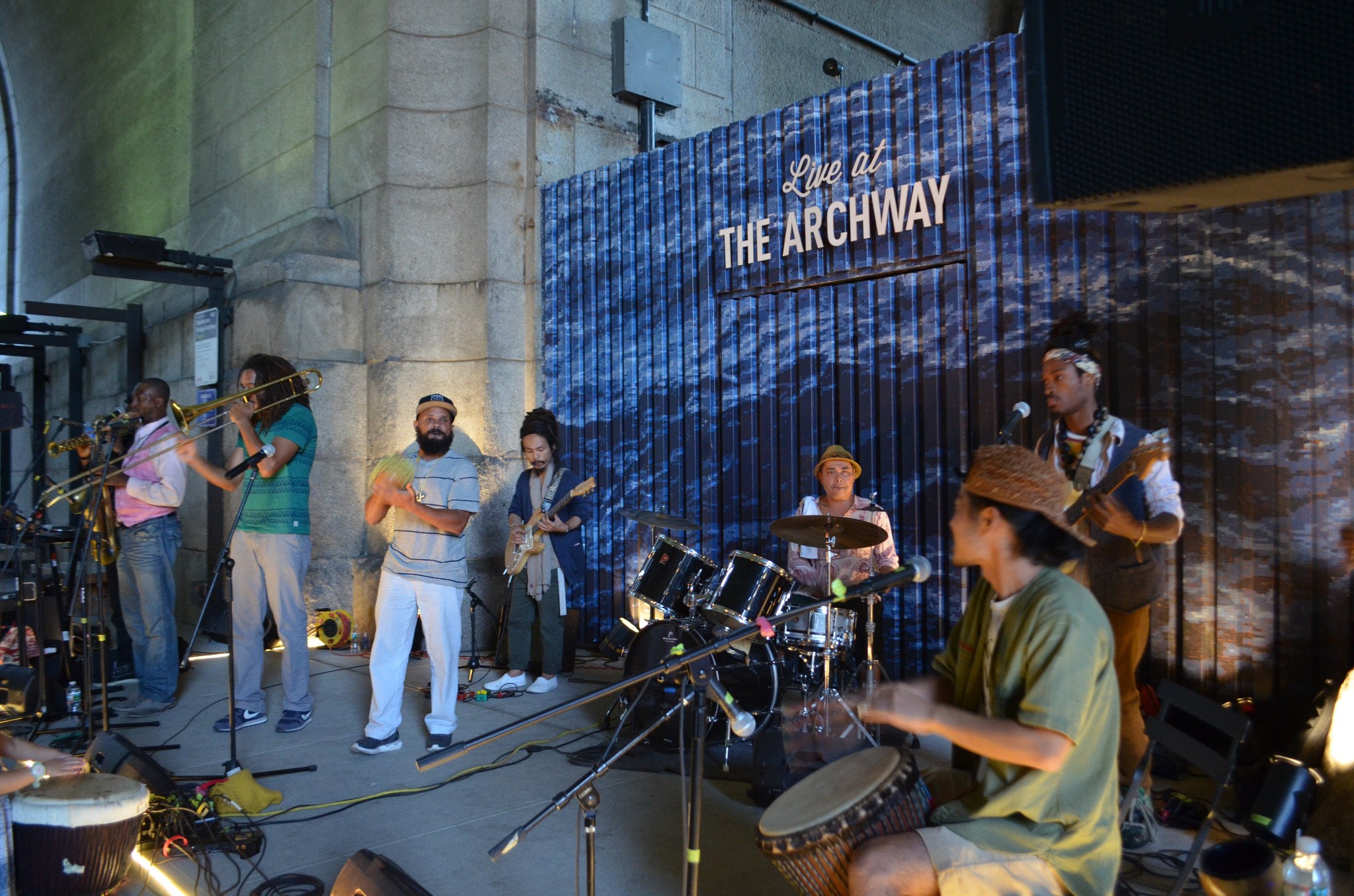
42 474 78 506
224 444 276 481
106 416 145 428
854 556 932 596
99 406 126 425
53 416 91 430
706 677 756 738
995 402 1031 445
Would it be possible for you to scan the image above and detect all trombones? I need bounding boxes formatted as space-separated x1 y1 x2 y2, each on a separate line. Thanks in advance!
37 367 323 508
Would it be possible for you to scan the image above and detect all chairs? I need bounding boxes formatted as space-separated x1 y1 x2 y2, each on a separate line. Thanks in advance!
1121 675 1253 896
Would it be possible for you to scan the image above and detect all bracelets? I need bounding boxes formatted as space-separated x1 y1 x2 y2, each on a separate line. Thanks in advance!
78 456 89 460
12 736 18 759
509 524 515 530
1132 520 1147 547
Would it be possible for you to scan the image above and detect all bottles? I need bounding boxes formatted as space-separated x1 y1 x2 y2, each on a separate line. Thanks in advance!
65 681 82 720
362 632 370 651
349 625 361 654
1282 835 1332 896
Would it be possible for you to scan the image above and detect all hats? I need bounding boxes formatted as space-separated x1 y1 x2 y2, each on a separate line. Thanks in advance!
415 393 457 425
964 445 1097 548
813 445 861 481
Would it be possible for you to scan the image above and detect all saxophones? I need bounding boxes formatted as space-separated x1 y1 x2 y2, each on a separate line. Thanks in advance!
78 412 133 565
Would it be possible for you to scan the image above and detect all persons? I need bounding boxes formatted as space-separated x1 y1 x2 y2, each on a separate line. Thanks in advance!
350 394 478 755
0 730 87 896
1036 327 1187 816
178 353 317 732
847 443 1123 896
76 377 189 717
787 445 899 688
483 408 589 694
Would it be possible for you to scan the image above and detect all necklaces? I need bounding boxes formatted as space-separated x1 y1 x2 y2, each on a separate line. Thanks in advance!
416 455 442 503
1058 406 1108 479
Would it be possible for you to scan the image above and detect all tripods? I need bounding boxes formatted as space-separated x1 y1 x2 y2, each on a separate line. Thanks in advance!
458 579 511 682
0 427 317 787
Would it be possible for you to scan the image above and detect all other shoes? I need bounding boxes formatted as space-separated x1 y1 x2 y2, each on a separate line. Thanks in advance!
526 676 558 693
484 672 526 691
1121 795 1155 848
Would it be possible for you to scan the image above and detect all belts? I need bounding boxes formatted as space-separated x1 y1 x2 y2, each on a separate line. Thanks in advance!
120 511 177 528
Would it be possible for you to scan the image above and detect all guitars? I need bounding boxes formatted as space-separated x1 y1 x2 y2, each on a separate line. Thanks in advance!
1058 428 1176 525
504 477 597 576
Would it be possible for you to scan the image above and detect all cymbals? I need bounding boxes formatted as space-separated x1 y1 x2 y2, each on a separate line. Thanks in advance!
619 505 703 531
769 515 888 549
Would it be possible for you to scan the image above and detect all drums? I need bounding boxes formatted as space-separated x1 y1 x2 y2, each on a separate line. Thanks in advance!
782 601 857 651
628 532 717 616
705 548 796 628
8 771 150 896
624 619 784 746
754 745 939 895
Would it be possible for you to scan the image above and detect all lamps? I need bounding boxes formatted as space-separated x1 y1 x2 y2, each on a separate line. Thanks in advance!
0 314 83 336
1244 751 1324 841
80 230 234 267
598 617 638 657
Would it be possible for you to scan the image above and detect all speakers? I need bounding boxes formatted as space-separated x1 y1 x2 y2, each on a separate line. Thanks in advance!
0 664 65 724
80 730 176 798
328 849 435 896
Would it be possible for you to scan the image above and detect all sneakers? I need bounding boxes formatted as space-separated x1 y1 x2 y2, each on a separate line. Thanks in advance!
213 708 267 732
128 697 177 718
351 728 402 754
427 733 452 752
275 708 312 733
110 693 148 711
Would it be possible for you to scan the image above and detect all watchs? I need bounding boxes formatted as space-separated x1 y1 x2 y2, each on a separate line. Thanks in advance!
31 761 47 780
564 524 570 533
